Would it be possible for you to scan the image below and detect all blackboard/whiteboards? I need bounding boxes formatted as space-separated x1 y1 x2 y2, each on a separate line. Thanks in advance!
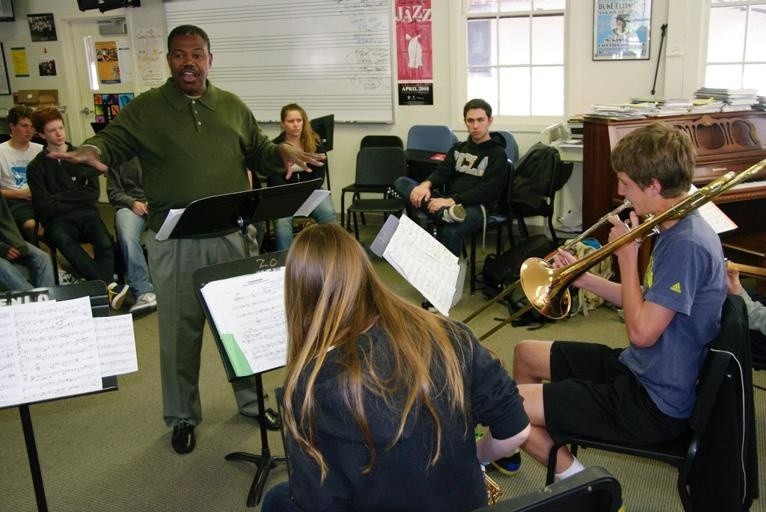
161 0 398 125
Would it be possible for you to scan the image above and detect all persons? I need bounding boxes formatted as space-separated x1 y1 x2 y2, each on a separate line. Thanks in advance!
105 156 158 318
262 102 338 253
726 268 766 339
391 99 507 313
0 106 44 245
258 223 531 512
489 122 726 512
0 187 55 292
45 24 328 456
405 20 424 80
613 17 629 36
26 107 130 312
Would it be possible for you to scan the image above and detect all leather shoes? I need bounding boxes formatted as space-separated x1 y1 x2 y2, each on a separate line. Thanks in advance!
239 408 282 431
171 423 196 454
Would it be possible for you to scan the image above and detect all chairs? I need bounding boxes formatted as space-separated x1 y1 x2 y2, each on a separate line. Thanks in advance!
346 146 407 242
30 187 93 286
462 158 516 294
341 136 404 229
469 465 623 512
496 150 560 254
546 294 746 512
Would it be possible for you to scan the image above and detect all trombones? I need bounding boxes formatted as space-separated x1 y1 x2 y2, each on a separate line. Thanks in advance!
461 157 766 341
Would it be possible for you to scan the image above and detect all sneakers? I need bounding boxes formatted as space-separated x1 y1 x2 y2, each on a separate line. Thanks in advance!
129 293 157 314
59 271 78 285
474 431 522 476
107 282 130 311
441 203 467 224
618 498 626 512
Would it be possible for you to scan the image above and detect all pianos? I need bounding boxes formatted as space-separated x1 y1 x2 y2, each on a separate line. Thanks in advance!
582 104 766 287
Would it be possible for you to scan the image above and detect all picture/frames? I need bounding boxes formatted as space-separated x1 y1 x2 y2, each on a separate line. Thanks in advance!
0 42 12 95
0 0 15 22
26 12 57 42
592 0 652 62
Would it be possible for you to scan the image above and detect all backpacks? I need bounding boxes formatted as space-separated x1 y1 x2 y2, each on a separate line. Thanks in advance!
563 237 616 319
510 140 560 210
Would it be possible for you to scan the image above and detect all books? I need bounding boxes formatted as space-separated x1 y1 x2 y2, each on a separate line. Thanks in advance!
567 84 766 140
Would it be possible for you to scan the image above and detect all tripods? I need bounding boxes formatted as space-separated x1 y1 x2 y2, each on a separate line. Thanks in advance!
191 217 288 508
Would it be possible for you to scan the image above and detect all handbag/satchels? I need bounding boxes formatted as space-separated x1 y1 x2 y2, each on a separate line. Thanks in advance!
503 284 556 331
474 234 558 302
